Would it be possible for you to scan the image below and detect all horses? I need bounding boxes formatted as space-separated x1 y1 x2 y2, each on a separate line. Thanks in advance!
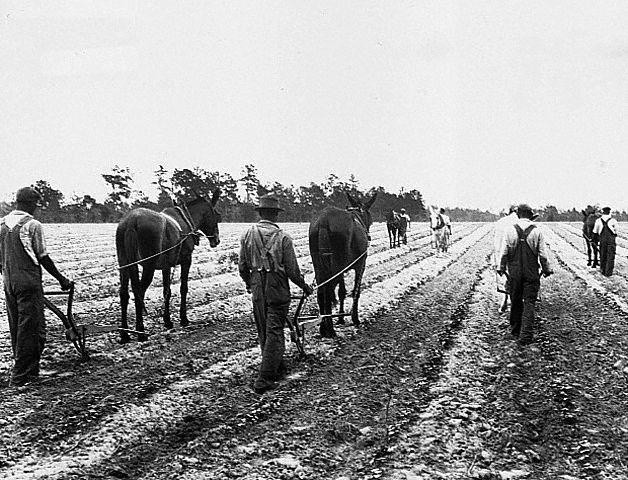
382 208 407 249
427 205 450 258
309 191 377 338
582 209 603 268
116 188 221 349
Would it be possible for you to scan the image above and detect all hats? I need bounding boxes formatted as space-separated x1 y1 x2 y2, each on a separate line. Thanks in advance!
601 206 612 211
517 203 533 213
254 194 285 212
15 185 40 203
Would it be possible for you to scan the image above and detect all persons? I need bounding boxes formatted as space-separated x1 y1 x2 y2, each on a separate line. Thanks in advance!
439 205 451 230
592 207 620 277
499 204 555 344
0 186 74 388
237 194 314 393
396 207 411 245
